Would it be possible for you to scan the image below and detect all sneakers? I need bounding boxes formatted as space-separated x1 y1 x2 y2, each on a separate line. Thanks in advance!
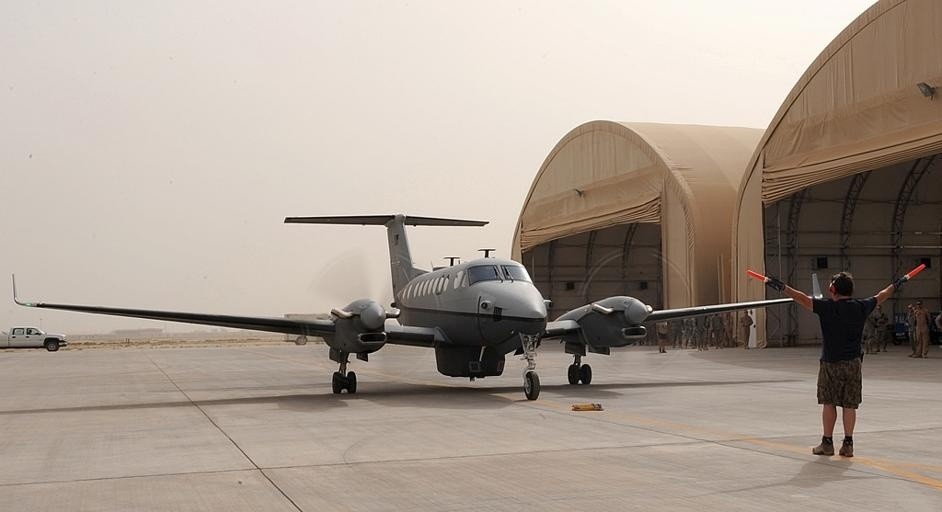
839 445 853 456
812 444 834 455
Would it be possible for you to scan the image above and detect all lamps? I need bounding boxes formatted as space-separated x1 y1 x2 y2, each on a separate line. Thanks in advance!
916 82 936 101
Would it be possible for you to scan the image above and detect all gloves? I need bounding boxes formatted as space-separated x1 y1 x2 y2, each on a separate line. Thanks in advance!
766 273 785 292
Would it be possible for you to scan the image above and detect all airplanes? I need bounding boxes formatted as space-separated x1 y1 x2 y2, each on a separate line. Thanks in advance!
12 214 824 399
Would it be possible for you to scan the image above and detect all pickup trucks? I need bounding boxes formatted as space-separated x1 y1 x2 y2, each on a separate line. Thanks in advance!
0 327 67 352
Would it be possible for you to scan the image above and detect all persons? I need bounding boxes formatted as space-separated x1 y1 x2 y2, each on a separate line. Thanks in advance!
861 313 881 356
656 319 669 354
739 309 754 350
935 313 941 328
764 270 909 458
912 300 933 359
671 312 738 352
905 302 918 359
873 304 890 354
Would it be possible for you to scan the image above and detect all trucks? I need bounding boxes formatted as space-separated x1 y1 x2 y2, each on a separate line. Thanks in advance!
283 313 332 345
